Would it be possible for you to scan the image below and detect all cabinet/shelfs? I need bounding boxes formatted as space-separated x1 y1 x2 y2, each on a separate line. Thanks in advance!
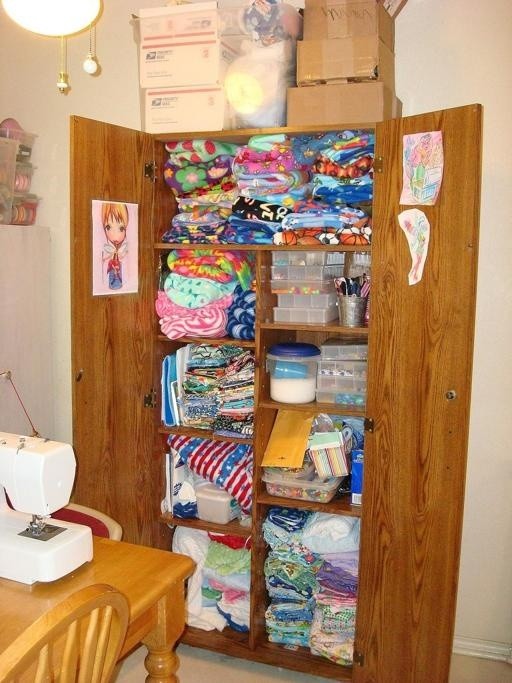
52 104 485 683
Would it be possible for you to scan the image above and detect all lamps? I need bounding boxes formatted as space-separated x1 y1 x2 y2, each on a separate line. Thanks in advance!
1 0 101 94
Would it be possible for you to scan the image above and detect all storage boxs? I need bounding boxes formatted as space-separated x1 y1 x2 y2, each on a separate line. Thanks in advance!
136 0 227 135
195 485 242 524
316 337 372 406
288 1 403 125
268 250 346 324
260 448 352 505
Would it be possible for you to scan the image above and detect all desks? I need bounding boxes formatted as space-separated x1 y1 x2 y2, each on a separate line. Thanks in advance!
0 534 198 683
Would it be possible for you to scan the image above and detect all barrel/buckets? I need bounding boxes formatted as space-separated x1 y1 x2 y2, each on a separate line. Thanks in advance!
336 294 367 326
266 343 321 403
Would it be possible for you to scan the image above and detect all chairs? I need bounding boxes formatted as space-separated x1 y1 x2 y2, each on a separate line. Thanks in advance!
52 504 123 543
0 584 131 683
